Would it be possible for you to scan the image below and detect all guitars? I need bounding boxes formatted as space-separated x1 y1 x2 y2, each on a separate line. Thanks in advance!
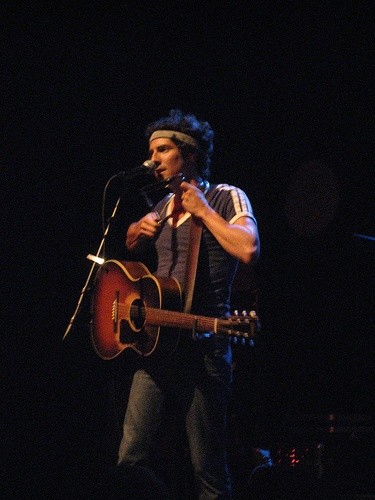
90 260 263 361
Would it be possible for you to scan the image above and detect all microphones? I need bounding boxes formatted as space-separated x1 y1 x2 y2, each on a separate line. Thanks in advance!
118 160 156 181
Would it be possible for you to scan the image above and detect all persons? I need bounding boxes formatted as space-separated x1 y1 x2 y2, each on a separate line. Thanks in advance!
116 110 261 500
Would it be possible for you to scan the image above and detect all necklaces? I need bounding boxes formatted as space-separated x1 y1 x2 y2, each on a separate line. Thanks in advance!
156 181 206 224
